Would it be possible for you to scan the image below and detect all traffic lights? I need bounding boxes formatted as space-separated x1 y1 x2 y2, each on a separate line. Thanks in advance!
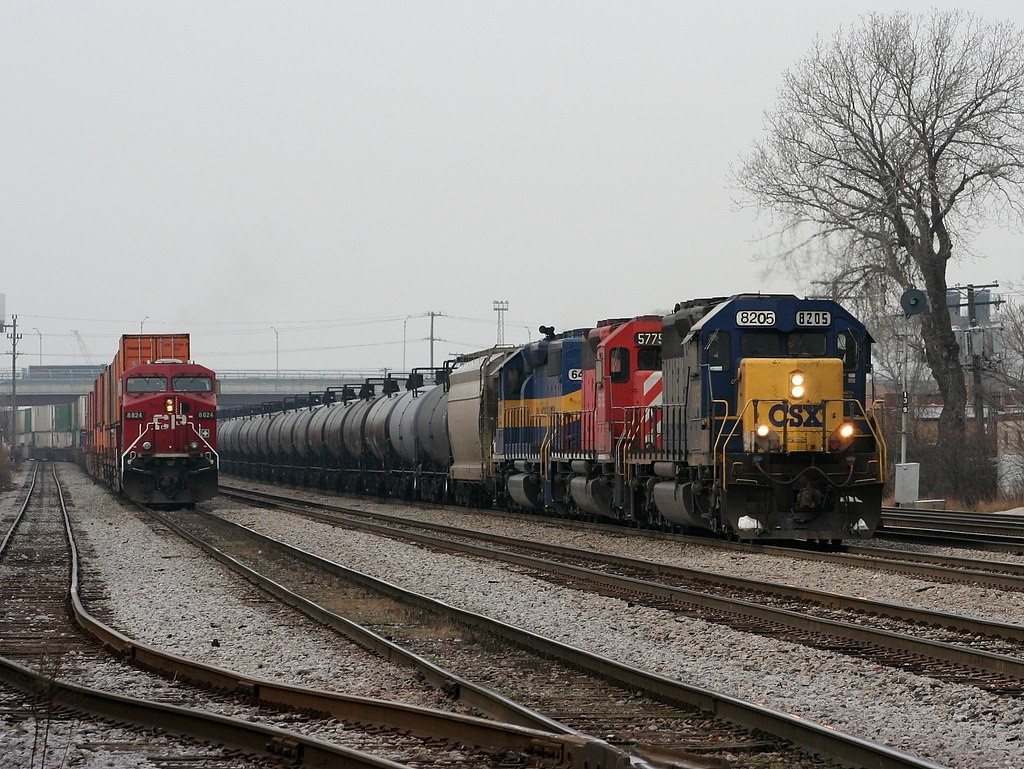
909 297 919 305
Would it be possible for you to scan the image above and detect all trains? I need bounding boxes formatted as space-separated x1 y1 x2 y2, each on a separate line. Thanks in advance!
217 292 888 552
0 334 219 510
